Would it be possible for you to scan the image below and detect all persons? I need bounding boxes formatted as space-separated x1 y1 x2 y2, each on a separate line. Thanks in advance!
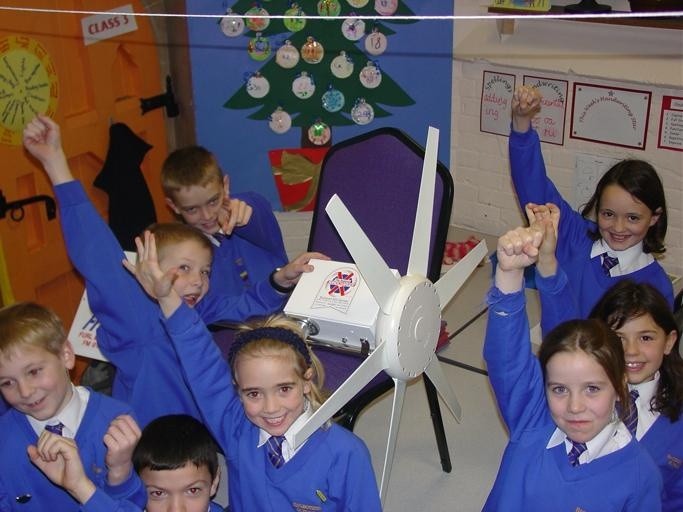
482 86 681 511
1 112 384 511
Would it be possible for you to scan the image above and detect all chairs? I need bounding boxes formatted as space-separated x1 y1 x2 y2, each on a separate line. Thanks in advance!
207 127 455 472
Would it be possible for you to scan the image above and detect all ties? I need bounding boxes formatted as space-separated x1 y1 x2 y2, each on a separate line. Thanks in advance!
565 437 588 468
266 436 288 471
599 252 619 278
45 423 64 437
213 232 228 246
622 390 639 438
